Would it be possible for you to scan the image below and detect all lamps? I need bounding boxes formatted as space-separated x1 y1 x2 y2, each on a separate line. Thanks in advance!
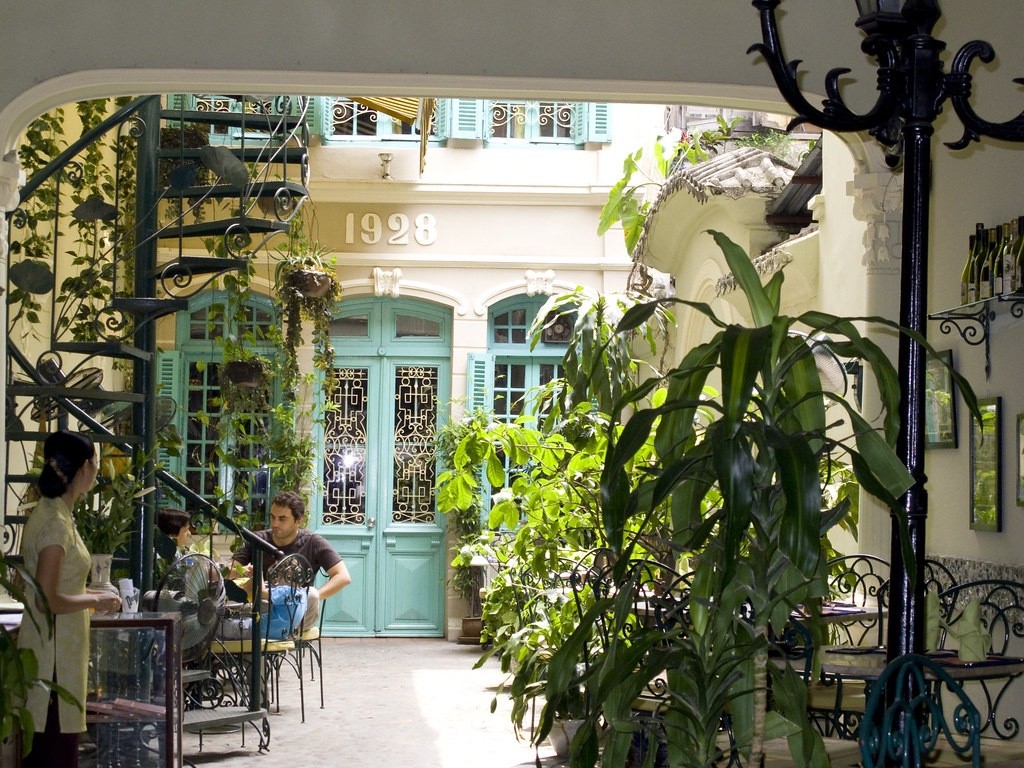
378 153 394 180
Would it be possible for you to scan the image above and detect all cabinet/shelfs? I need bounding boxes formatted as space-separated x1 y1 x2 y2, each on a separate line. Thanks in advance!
78 610 184 768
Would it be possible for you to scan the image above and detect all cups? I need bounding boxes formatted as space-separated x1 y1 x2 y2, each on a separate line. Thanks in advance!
121 588 140 614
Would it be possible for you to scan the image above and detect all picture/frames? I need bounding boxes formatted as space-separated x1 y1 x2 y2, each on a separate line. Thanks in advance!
967 396 1004 534
1016 413 1024 507
925 348 959 451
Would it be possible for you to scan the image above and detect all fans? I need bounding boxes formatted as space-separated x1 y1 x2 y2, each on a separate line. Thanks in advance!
141 554 227 768
122 395 178 437
31 358 103 422
77 382 134 434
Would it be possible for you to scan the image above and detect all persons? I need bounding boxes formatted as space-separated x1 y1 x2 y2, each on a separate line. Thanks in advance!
233 490 351 715
154 506 254 603
4 429 123 768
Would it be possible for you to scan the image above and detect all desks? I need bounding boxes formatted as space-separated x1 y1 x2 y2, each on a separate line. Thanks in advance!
633 599 889 636
771 645 1024 768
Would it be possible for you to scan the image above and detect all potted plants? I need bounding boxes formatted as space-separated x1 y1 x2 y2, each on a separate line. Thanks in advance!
471 580 643 759
189 228 344 574
0 623 84 768
24 440 166 596
439 566 484 645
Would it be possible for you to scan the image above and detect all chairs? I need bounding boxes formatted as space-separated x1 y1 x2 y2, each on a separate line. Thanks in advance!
184 553 331 746
506 547 1024 768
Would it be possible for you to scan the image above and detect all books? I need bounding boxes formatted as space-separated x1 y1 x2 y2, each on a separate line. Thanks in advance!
790 601 866 617
